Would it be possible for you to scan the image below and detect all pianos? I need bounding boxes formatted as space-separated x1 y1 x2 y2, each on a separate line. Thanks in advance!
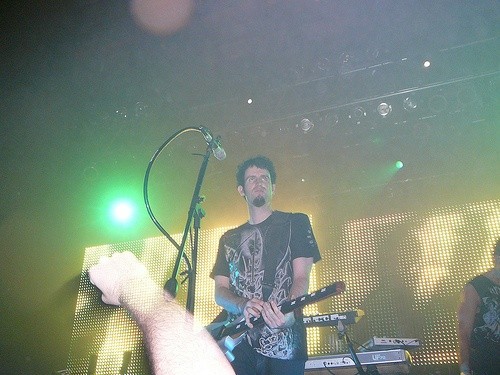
356 336 419 375
303 310 407 375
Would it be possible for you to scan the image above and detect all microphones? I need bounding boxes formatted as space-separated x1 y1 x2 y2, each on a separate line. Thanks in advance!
200 126 226 161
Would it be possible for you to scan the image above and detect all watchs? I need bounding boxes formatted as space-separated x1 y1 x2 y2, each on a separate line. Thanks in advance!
459 364 471 371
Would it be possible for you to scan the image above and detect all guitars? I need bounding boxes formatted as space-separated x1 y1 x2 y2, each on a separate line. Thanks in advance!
204 282 346 362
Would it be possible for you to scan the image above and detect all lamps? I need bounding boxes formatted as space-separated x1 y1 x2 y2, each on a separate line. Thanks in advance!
80 41 500 178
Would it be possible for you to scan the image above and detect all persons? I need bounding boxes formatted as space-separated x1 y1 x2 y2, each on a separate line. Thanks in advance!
212 157 322 375
458 239 500 375
88 251 236 375
